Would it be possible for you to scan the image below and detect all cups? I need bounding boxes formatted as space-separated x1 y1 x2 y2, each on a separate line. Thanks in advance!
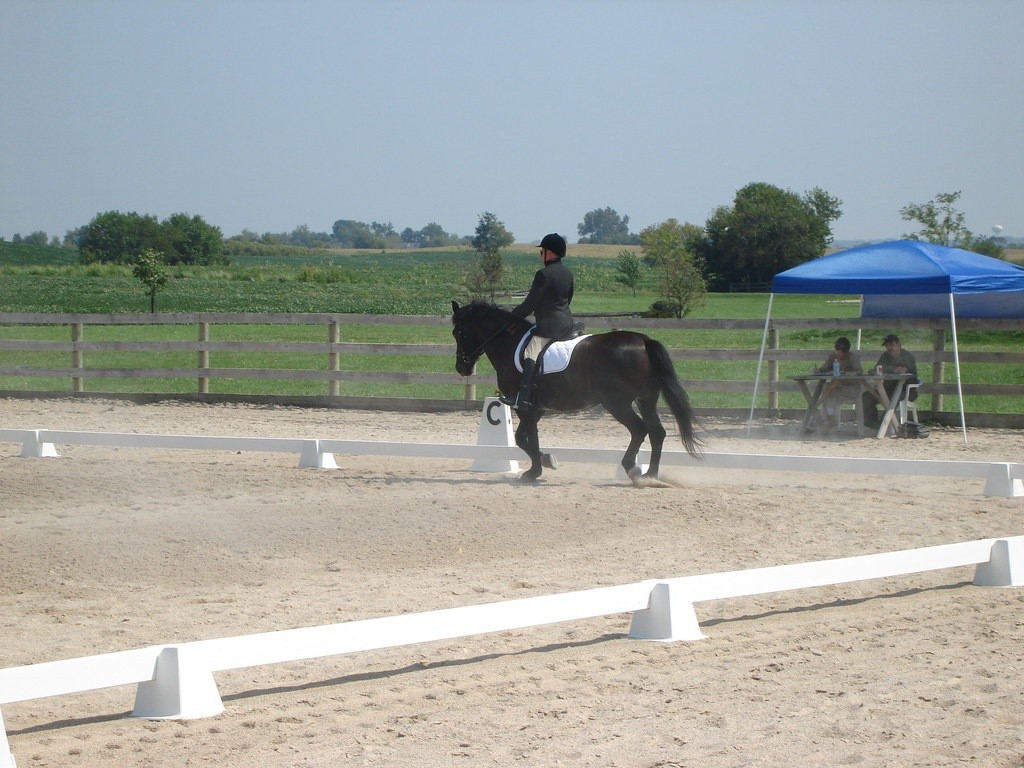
876 366 883 376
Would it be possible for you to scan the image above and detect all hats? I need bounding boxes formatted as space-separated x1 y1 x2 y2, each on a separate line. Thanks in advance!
882 335 898 346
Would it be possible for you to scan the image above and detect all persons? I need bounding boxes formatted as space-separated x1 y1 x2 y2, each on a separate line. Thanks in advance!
795 337 863 435
862 335 917 439
498 234 574 411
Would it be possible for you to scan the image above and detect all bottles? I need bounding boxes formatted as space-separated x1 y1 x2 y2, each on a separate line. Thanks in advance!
833 359 840 376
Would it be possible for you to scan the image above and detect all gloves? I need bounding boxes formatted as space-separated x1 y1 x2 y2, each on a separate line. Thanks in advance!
508 314 519 321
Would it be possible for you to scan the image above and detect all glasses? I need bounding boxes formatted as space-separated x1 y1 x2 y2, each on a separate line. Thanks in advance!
540 251 544 255
836 342 846 349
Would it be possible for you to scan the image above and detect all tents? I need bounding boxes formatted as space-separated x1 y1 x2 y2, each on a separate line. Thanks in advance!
746 239 1024 443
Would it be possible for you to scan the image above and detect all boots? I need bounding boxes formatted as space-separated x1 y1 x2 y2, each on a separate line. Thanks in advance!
498 358 539 408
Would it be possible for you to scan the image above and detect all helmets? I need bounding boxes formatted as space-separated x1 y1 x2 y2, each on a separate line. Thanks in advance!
536 233 566 257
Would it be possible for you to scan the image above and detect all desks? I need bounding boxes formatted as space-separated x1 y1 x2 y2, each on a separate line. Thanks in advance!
784 373 917 440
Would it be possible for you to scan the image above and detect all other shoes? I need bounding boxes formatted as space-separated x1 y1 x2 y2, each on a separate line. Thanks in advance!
861 427 879 437
820 415 836 435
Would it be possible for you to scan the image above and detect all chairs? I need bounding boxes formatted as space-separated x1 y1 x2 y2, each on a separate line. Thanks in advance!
807 381 864 433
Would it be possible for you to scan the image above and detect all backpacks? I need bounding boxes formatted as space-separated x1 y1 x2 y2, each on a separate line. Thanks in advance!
897 421 930 439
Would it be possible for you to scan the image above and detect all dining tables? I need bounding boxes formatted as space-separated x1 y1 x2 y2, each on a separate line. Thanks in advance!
854 379 923 437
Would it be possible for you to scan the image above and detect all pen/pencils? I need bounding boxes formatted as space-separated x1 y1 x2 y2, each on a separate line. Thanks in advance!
814 364 818 370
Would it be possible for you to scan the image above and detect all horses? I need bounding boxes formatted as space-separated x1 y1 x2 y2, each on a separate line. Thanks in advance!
449 297 712 484
